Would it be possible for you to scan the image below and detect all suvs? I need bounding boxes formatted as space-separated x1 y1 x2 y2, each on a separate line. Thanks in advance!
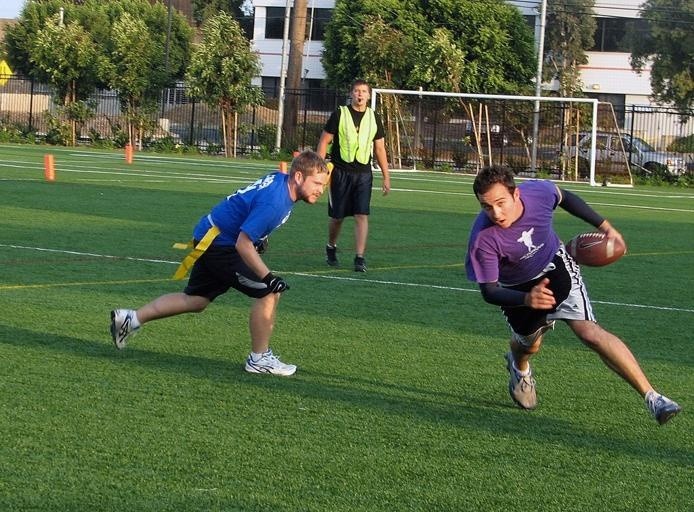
555 129 688 183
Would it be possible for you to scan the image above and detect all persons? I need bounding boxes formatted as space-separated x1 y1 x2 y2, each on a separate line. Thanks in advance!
465 164 682 426
110 150 330 376
317 80 390 272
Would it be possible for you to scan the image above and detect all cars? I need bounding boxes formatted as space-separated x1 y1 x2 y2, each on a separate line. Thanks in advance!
469 110 535 148
465 120 474 136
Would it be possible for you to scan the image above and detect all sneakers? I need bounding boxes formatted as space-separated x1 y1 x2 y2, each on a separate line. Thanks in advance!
326 244 367 272
110 308 135 350
503 352 537 410
243 349 297 376
648 394 683 425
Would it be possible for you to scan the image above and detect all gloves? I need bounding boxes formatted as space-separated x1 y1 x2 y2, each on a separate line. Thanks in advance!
260 271 290 293
253 235 269 257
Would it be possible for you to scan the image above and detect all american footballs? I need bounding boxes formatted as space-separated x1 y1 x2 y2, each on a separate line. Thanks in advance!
565 233 625 267
